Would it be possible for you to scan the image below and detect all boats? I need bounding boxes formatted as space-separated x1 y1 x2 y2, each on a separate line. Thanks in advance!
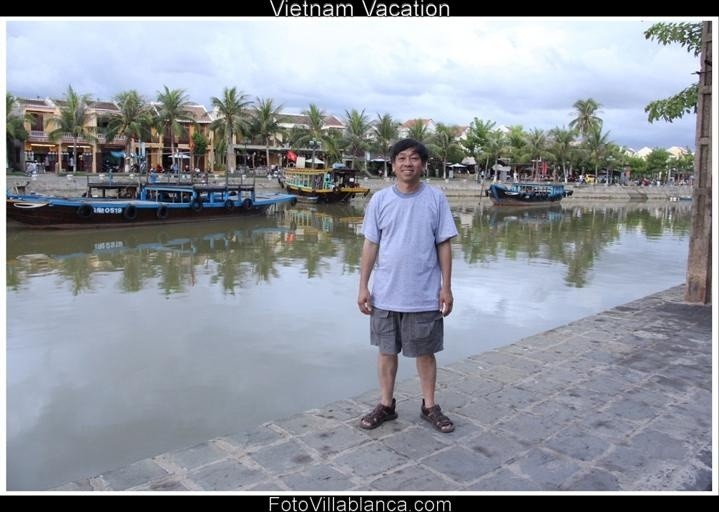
485 181 565 206
277 167 371 204
7 181 298 224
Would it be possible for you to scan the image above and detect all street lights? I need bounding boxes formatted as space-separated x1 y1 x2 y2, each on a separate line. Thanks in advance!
309 138 321 168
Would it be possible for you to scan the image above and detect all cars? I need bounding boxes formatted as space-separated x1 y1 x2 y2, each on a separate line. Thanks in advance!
583 174 597 183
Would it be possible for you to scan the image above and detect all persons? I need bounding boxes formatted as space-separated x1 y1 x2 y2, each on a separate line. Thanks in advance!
355 138 459 433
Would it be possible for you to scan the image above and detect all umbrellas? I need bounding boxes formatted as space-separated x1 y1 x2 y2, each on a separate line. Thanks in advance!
450 162 467 168
169 152 191 160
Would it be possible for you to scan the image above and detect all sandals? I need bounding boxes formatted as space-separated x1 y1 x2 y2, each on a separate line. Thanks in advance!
420 398 455 433
360 398 398 429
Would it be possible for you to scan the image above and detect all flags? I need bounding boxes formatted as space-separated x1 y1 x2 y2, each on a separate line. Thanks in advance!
287 151 297 161
